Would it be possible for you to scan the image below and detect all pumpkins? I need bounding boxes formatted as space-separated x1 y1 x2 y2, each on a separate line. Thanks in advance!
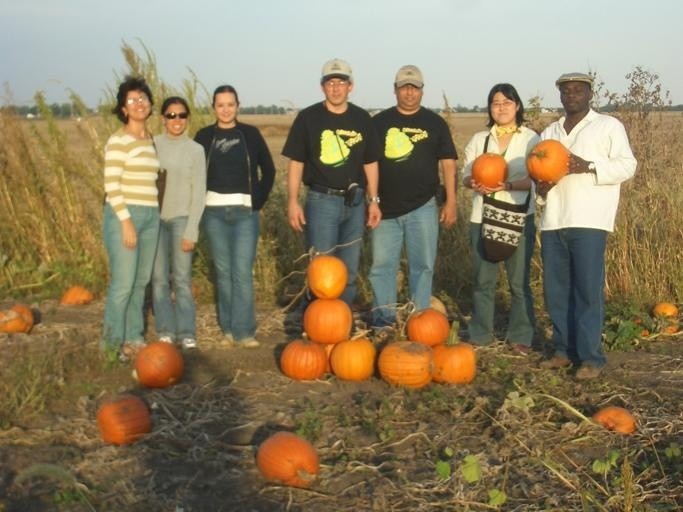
632 318 648 335
407 308 450 347
0 306 34 334
324 344 335 373
97 393 151 444
60 285 94 306
304 298 352 343
330 338 376 381
592 406 637 434
135 342 185 388
525 140 571 183
281 333 327 381
308 255 348 298
378 342 433 389
653 303 680 333
257 431 319 489
471 154 509 189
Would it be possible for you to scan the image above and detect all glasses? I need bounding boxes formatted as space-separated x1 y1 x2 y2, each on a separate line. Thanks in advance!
164 112 189 120
321 81 345 86
127 95 148 107
489 102 513 106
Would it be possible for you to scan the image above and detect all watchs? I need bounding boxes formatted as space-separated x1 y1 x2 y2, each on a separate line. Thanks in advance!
368 197 380 204
589 161 595 173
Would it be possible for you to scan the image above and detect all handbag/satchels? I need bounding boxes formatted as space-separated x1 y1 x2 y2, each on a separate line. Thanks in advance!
479 130 531 263
102 132 167 212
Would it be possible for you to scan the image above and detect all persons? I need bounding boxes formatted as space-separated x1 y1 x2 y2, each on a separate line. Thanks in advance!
280 58 382 336
194 84 277 347
537 73 638 380
151 97 207 351
462 83 541 358
101 76 162 363
363 65 458 333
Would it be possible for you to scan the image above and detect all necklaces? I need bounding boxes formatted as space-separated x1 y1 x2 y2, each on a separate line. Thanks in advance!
497 126 517 137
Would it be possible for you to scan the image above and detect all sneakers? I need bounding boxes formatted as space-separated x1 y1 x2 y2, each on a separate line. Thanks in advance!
224 333 236 342
111 352 129 360
574 363 602 380
240 336 262 347
181 338 198 352
508 340 528 354
124 341 146 351
374 331 394 339
537 358 571 368
158 335 174 343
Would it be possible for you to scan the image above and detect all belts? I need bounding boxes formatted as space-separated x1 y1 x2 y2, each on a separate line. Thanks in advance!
308 183 369 197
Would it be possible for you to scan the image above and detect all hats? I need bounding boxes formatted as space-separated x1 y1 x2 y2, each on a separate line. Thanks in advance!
393 65 425 88
319 59 352 82
554 72 595 87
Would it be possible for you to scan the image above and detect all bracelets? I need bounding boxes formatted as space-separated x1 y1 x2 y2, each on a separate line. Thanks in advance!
507 182 513 190
